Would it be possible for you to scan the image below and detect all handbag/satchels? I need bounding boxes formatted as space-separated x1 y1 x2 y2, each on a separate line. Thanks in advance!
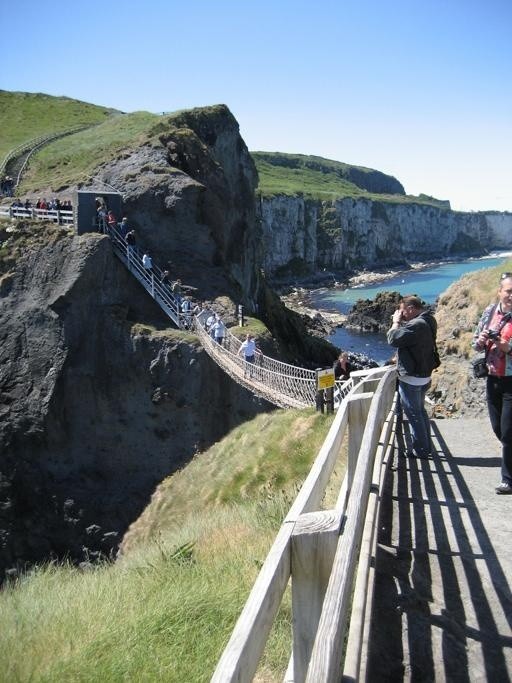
472 353 487 378
434 351 441 369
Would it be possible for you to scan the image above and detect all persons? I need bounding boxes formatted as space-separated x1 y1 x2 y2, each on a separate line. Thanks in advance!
142 250 153 280
333 352 351 381
95 199 136 260
0 176 14 197
386 294 437 458
472 272 511 492
173 279 182 302
161 271 170 284
180 297 225 345
10 197 73 224
236 334 262 379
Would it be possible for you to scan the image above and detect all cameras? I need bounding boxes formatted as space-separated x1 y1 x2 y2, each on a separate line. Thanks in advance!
489 330 498 340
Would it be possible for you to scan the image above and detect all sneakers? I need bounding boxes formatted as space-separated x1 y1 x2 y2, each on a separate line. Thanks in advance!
494 482 512 493
402 449 428 458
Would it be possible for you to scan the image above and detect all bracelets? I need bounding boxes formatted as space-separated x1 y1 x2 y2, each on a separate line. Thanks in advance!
392 320 401 324
